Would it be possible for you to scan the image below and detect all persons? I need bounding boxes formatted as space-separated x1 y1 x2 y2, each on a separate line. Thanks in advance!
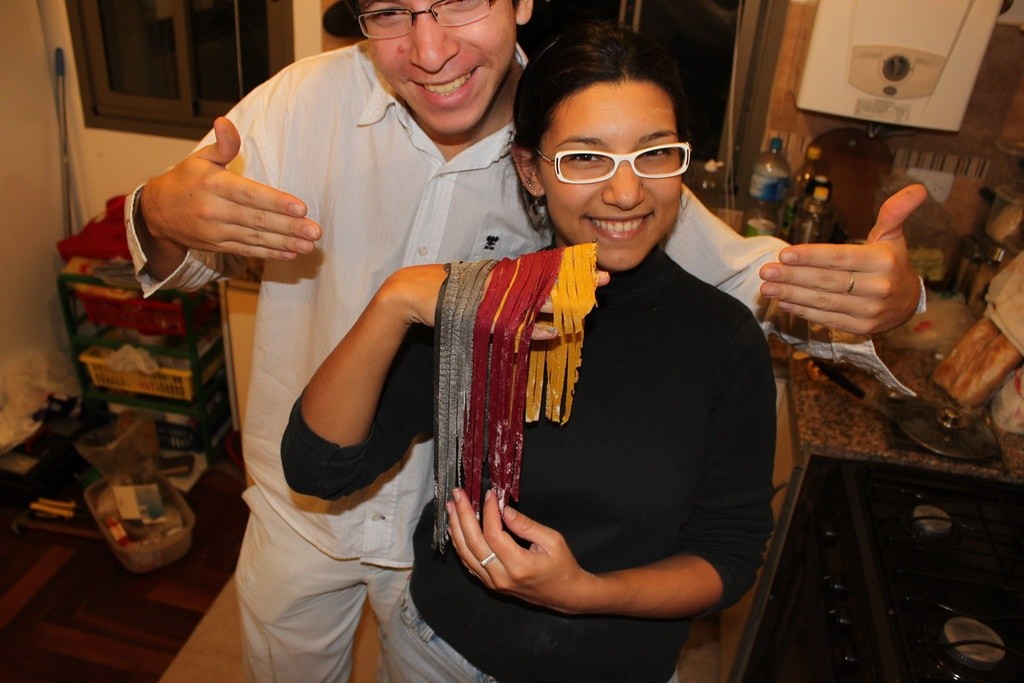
281 19 779 683
124 0 926 683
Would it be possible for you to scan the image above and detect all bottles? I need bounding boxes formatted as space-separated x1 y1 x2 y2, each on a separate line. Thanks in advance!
750 139 791 213
788 176 837 243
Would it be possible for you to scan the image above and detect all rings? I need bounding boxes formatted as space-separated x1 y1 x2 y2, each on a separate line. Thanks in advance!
845 272 856 294
469 571 472 574
481 553 496 566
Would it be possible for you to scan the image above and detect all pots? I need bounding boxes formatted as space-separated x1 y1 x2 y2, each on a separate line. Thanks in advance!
813 358 1000 461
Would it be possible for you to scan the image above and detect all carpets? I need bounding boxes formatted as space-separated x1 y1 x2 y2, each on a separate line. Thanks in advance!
0 427 250 683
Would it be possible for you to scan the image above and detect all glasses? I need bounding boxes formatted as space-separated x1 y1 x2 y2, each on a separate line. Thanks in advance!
535 141 691 185
348 0 497 39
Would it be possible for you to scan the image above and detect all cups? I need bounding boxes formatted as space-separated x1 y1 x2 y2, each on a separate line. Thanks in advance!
746 218 777 237
717 208 743 234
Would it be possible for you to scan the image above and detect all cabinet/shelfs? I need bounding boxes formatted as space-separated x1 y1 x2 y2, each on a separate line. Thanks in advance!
214 278 262 433
720 378 795 683
57 270 229 469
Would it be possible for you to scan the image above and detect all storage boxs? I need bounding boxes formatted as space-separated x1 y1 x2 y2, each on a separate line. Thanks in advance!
53 399 196 574
985 182 1024 255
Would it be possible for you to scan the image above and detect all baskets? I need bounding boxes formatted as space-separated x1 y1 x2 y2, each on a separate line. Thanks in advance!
76 410 158 479
71 288 219 339
78 341 225 402
85 467 195 572
152 382 231 455
